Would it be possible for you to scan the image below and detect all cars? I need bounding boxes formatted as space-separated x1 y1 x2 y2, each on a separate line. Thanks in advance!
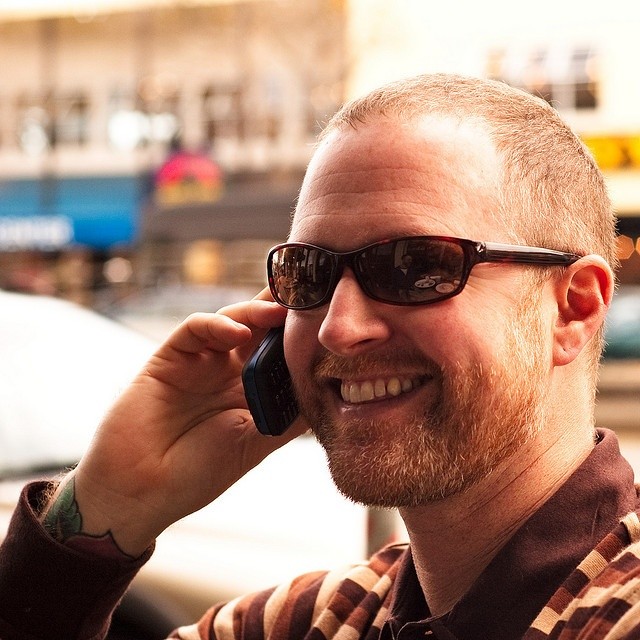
0 290 411 640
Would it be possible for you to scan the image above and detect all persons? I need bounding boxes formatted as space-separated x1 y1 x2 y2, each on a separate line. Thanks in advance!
388 255 412 301
0 72 640 640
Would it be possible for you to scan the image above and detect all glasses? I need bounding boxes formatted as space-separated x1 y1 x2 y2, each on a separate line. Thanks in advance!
264 234 581 311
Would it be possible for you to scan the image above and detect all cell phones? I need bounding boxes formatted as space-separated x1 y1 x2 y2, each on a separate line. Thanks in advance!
241 327 301 436
